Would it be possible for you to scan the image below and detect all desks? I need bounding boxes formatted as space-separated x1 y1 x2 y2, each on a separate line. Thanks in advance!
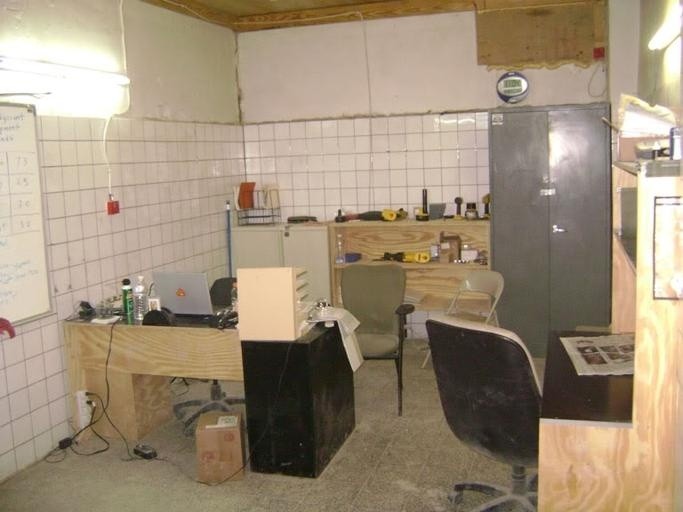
62 299 357 479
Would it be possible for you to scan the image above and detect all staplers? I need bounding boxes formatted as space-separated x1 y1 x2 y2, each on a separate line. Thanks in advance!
220 312 238 328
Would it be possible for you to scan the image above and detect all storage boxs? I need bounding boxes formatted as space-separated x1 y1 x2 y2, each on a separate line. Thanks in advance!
194 411 247 482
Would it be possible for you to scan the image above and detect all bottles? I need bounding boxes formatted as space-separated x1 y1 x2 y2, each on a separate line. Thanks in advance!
335 234 345 264
652 145 661 159
231 282 238 310
121 275 147 320
465 203 478 219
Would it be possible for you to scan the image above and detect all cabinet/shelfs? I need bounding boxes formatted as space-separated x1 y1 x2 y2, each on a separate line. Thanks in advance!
538 159 683 511
488 102 610 358
329 217 492 314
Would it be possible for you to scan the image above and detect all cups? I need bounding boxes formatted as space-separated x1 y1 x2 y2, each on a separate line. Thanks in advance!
671 128 681 160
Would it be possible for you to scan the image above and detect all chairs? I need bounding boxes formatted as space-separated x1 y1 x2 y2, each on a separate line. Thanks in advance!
338 263 415 416
419 269 504 370
425 316 542 510
171 276 246 439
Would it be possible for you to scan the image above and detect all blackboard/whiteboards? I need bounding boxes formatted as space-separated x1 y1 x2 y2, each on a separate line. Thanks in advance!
1 103 54 330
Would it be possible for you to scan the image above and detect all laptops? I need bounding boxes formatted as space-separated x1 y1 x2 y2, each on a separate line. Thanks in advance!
151 270 226 315
